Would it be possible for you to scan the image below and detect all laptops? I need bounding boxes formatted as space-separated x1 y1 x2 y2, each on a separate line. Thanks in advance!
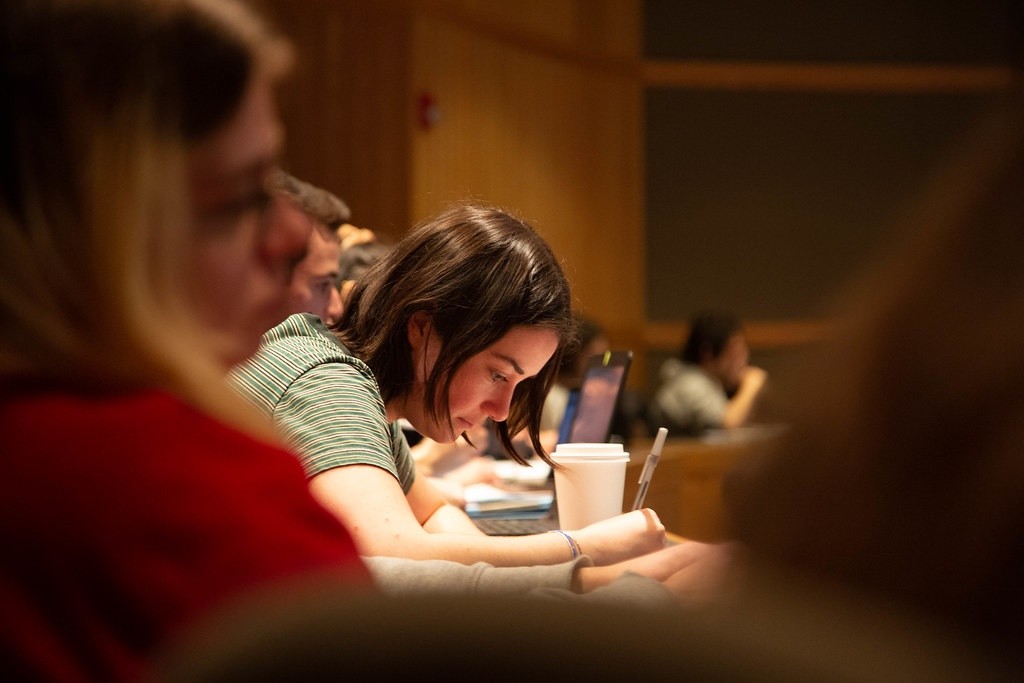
470 350 633 537
465 387 583 513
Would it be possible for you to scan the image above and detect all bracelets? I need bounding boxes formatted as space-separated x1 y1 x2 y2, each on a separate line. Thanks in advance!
551 529 583 562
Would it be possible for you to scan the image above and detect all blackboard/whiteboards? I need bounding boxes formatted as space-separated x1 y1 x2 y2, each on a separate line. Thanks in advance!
644 58 1010 350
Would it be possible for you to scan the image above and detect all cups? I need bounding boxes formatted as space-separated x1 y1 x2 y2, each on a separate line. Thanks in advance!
550 442 631 532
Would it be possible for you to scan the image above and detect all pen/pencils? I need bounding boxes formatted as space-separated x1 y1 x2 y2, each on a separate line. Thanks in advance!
632 426 668 513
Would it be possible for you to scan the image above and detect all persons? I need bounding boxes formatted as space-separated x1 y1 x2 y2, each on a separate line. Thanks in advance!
226 205 667 565
526 323 608 430
648 314 771 435
276 175 501 527
335 242 491 479
0 0 757 683
